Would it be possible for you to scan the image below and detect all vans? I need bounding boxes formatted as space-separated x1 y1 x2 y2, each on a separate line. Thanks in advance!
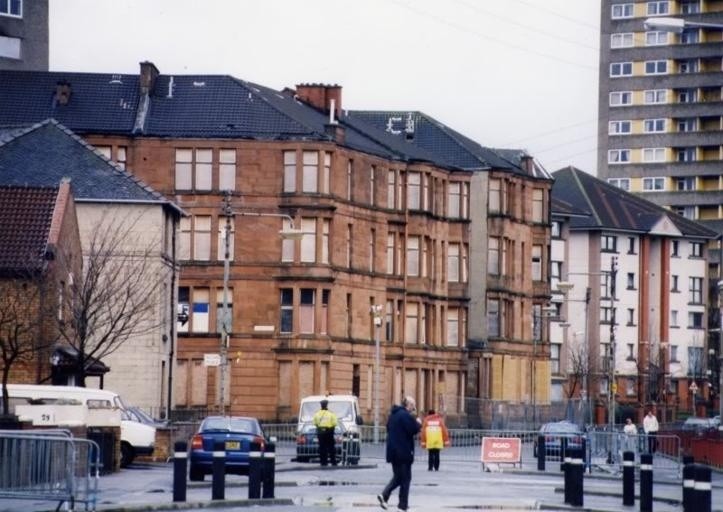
296 392 364 468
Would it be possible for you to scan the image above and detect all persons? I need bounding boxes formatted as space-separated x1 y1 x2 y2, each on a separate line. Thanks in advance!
377 396 423 512
643 409 659 456
312 400 339 466
622 419 636 433
420 408 450 471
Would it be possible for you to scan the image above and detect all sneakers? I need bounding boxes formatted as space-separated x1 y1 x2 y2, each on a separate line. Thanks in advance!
377 494 386 508
397 506 411 512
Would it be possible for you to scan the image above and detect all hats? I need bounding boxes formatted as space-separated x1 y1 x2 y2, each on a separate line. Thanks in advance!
321 400 328 404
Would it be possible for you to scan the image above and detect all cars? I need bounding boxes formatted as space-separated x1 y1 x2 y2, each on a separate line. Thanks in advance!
533 419 592 461
680 414 723 436
296 420 349 466
189 415 266 483
123 403 170 428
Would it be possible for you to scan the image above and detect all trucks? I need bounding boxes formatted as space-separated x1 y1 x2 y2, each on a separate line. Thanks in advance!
0 382 159 468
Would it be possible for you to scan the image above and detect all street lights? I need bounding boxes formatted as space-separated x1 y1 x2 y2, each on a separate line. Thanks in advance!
555 256 621 464
217 188 307 417
368 304 386 447
